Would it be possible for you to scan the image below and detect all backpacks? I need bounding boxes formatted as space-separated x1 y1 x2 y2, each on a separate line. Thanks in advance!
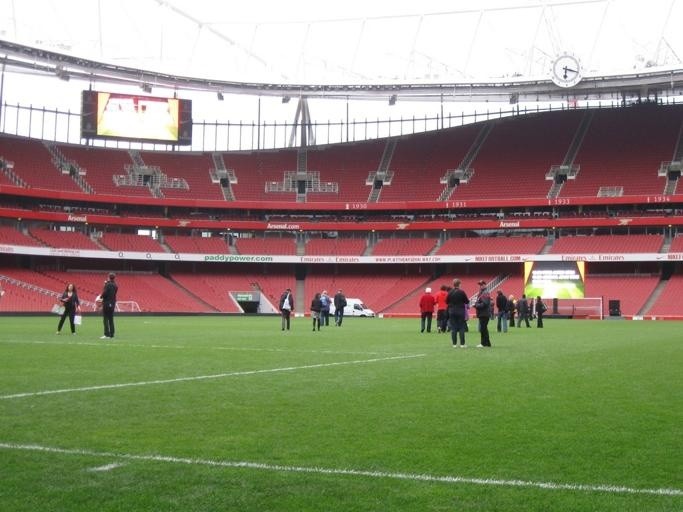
320 297 327 307
340 295 346 306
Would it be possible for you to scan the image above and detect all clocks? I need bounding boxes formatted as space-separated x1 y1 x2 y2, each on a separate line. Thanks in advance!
547 50 584 89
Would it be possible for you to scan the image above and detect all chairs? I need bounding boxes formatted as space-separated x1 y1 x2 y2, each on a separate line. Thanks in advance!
0 103 682 320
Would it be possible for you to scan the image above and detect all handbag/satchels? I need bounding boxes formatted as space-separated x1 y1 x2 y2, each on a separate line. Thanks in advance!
104 302 114 312
283 299 291 311
73 316 81 324
541 302 547 312
52 304 65 315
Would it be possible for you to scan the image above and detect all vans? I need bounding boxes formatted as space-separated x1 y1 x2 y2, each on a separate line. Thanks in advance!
328 297 375 317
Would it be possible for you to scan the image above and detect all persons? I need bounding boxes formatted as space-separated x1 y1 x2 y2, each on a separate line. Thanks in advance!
278 287 295 331
334 288 346 327
445 278 470 349
310 287 333 332
417 286 548 333
471 280 492 348
55 282 82 336
99 272 118 340
